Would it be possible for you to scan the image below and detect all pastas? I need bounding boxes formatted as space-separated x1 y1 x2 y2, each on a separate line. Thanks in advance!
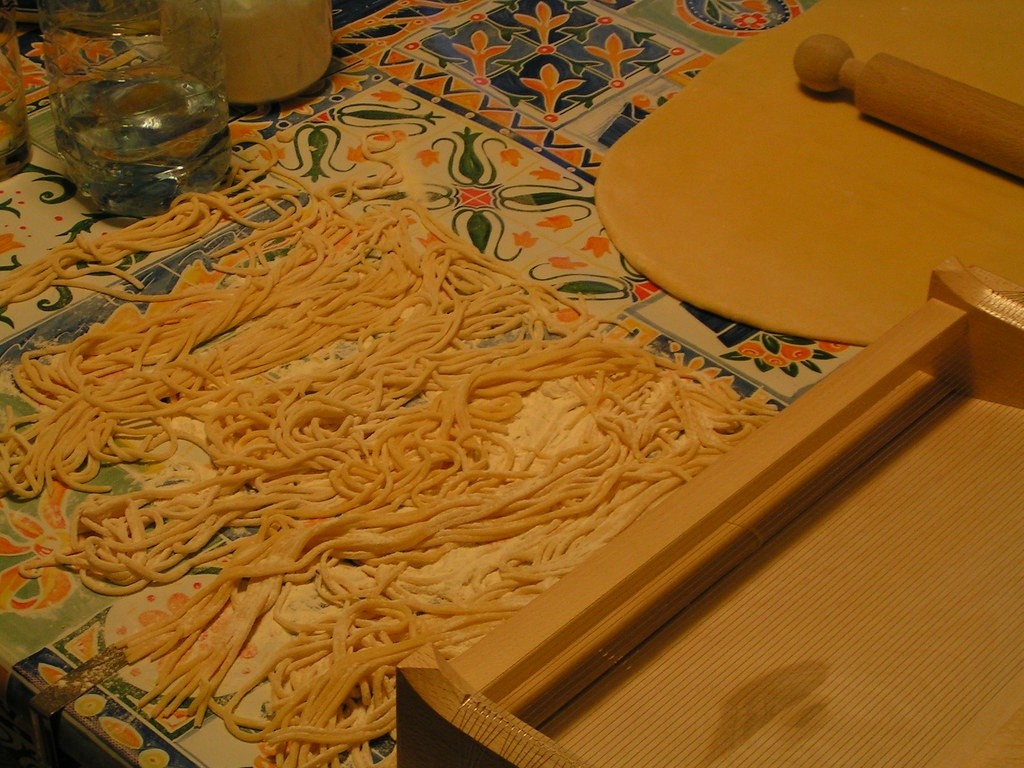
0 130 776 768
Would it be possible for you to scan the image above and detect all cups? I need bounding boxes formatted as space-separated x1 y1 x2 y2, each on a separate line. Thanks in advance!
37 0 232 218
0 9 34 182
159 0 334 108
